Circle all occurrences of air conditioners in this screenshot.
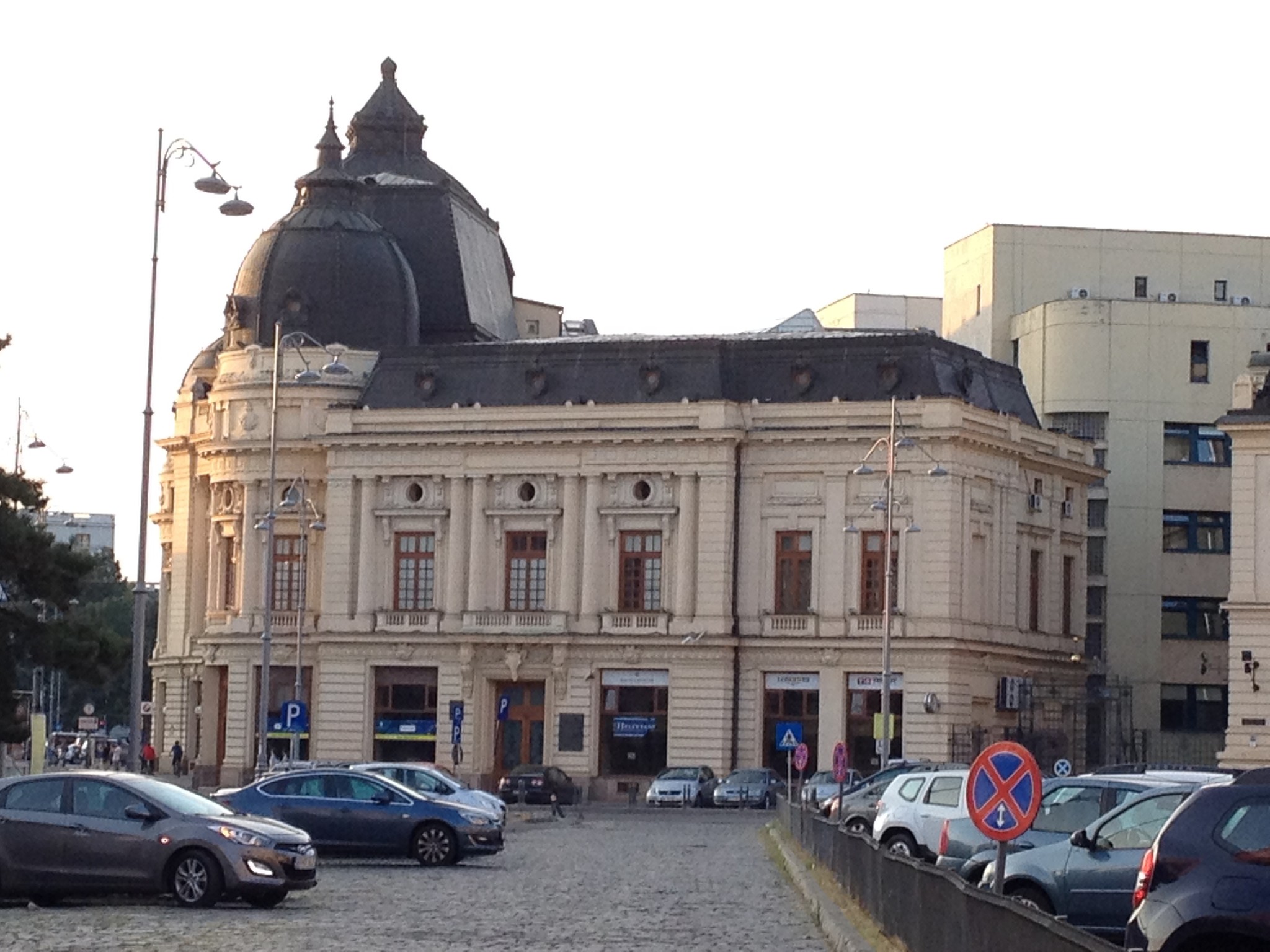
[1228,295,1253,306]
[1071,286,1090,299]
[1158,292,1178,302]
[1029,494,1043,509]
[998,675,1019,712]
[1062,501,1074,516]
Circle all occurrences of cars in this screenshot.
[871,771,971,857]
[831,779,893,837]
[984,785,1196,948]
[210,768,506,869]
[352,763,508,825]
[646,765,719,808]
[816,760,971,819]
[936,773,1162,887]
[713,768,787,809]
[801,769,865,804]
[0,770,318,909]
[496,762,580,805]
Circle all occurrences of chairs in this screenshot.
[76,790,122,817]
[318,778,354,800]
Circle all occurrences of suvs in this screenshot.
[1125,767,1270,952]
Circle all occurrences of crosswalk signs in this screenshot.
[776,722,802,750]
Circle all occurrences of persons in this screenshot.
[45,737,89,766]
[112,741,122,770]
[168,740,184,774]
[102,742,111,769]
[142,742,156,771]
[542,772,567,818]
[281,747,288,762]
[269,748,280,772]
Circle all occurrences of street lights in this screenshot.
[130,137,256,772]
[14,398,73,475]
[256,329,350,779]
[840,407,948,770]
[279,475,326,762]
